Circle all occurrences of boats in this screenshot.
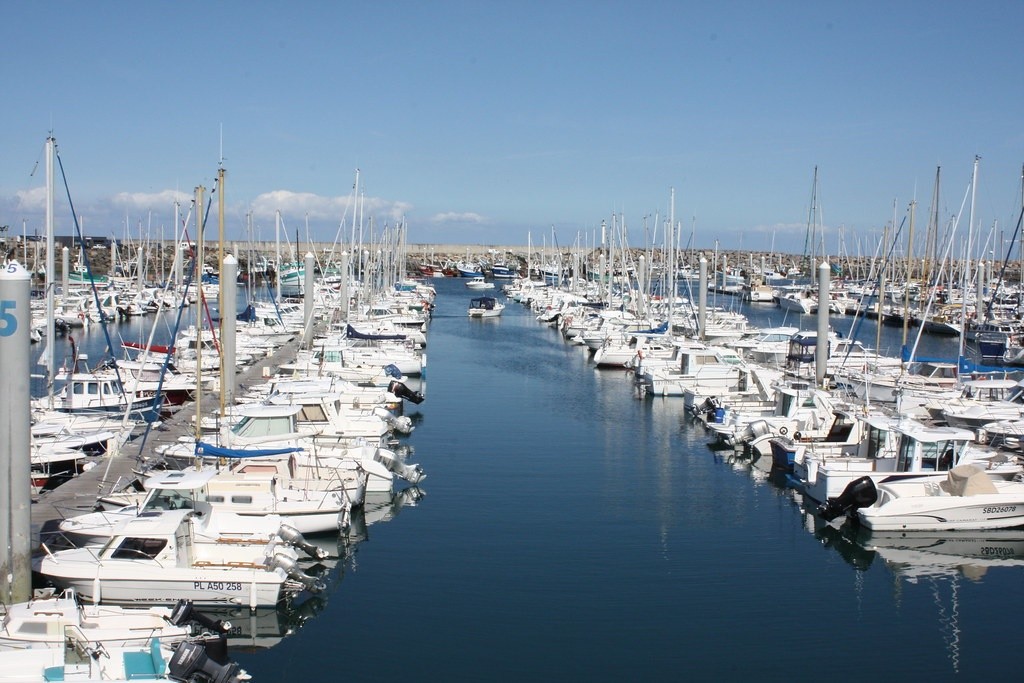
[466,276,495,289]
[0,587,252,682]
[467,296,505,320]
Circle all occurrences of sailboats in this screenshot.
[2,132,437,616]
[457,148,1024,538]
[419,246,458,278]
